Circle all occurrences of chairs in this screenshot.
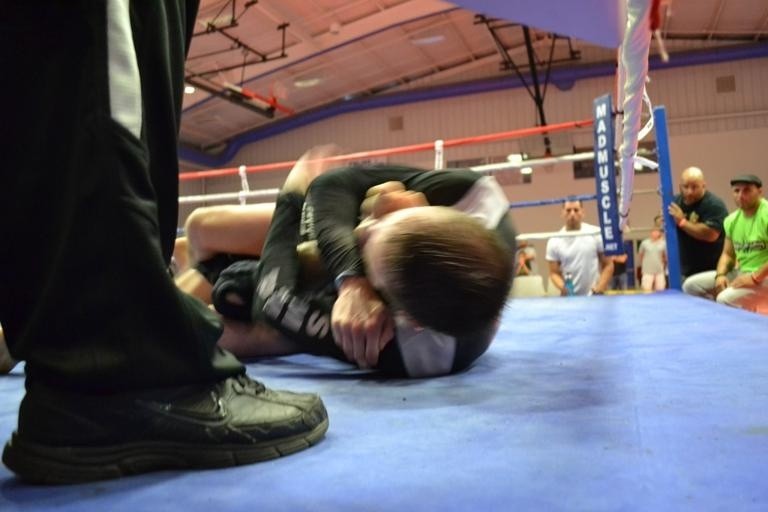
[547,274,562,295]
[509,275,544,296]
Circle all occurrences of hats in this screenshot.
[730,174,762,189]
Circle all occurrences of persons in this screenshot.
[606,249,628,290]
[680,174,768,318]
[544,194,614,296]
[514,239,538,276]
[514,251,529,276]
[638,230,667,291]
[666,166,729,297]
[0,1,329,488]
[171,143,517,381]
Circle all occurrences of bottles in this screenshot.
[565,273,573,297]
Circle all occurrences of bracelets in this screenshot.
[750,272,761,284]
[714,273,726,280]
[677,217,687,228]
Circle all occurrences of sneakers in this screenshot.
[2,367,332,486]
